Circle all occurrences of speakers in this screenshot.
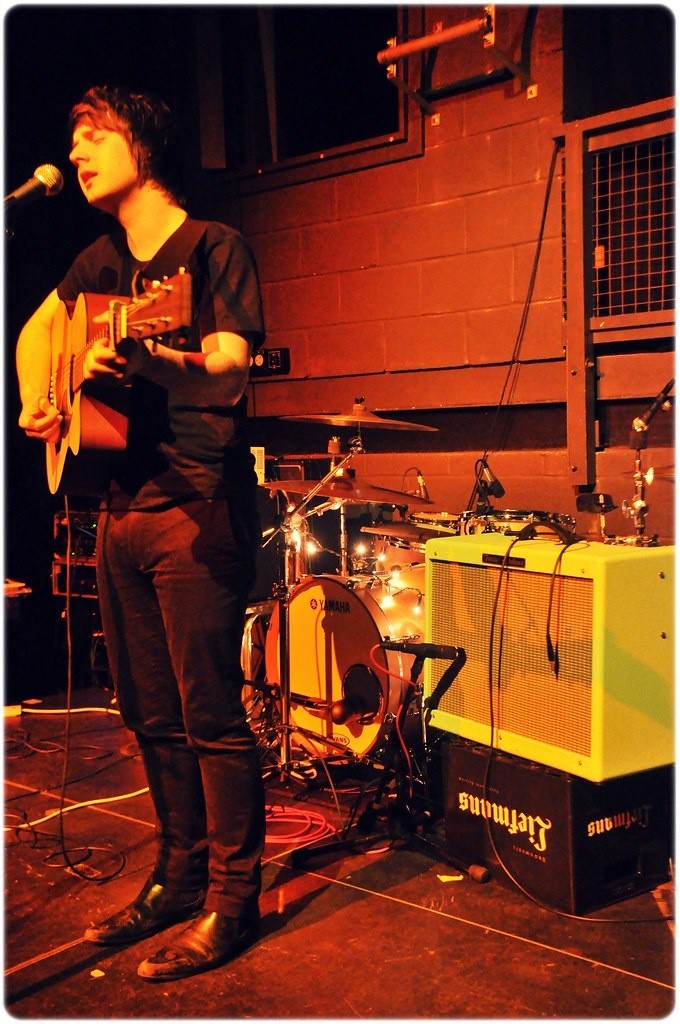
[423,532,676,783]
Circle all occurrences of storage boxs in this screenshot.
[432,732,675,917]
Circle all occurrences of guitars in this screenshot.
[43,265,196,498]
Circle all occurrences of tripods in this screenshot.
[240,419,397,791]
[290,655,489,884]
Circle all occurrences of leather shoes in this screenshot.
[85,885,207,947]
[136,910,261,981]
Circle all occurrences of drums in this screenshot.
[460,508,578,543]
[262,560,436,768]
[409,510,461,554]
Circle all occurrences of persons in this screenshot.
[13,81,268,983]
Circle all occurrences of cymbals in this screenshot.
[278,394,439,433]
[260,465,437,506]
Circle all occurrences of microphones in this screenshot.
[380,642,464,661]
[330,698,357,725]
[417,470,429,500]
[631,378,675,432]
[482,459,505,499]
[4,164,64,214]
